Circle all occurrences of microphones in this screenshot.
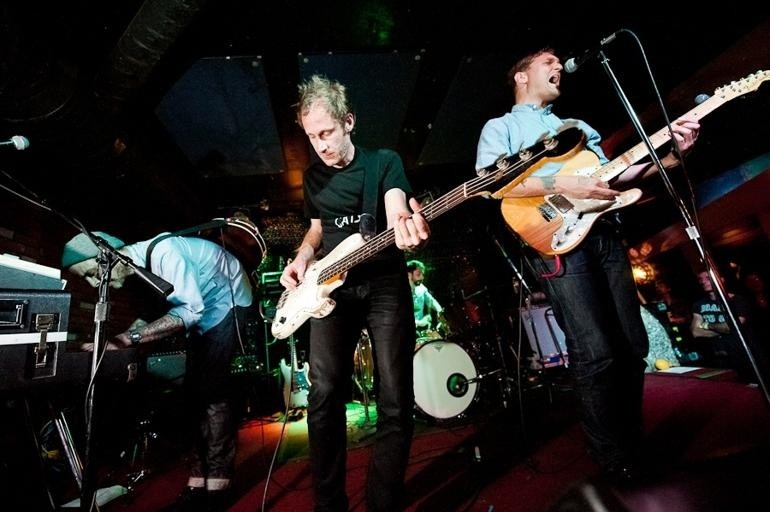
[456,375,480,391]
[564,26,622,73]
[0,132,31,154]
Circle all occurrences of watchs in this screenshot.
[128,328,143,346]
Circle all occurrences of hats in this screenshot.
[61,230,126,268]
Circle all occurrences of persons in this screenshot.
[655,278,697,351]
[279,71,438,511]
[404,258,443,336]
[684,259,757,383]
[473,45,704,500]
[60,230,257,512]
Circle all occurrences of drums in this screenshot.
[443,300,487,337]
[351,329,377,394]
[412,335,484,427]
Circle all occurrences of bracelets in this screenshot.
[669,143,693,161]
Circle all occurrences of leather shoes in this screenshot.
[174,474,239,512]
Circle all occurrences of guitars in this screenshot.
[269,124,588,341]
[501,69,770,256]
[279,334,313,410]
[191,216,267,289]
